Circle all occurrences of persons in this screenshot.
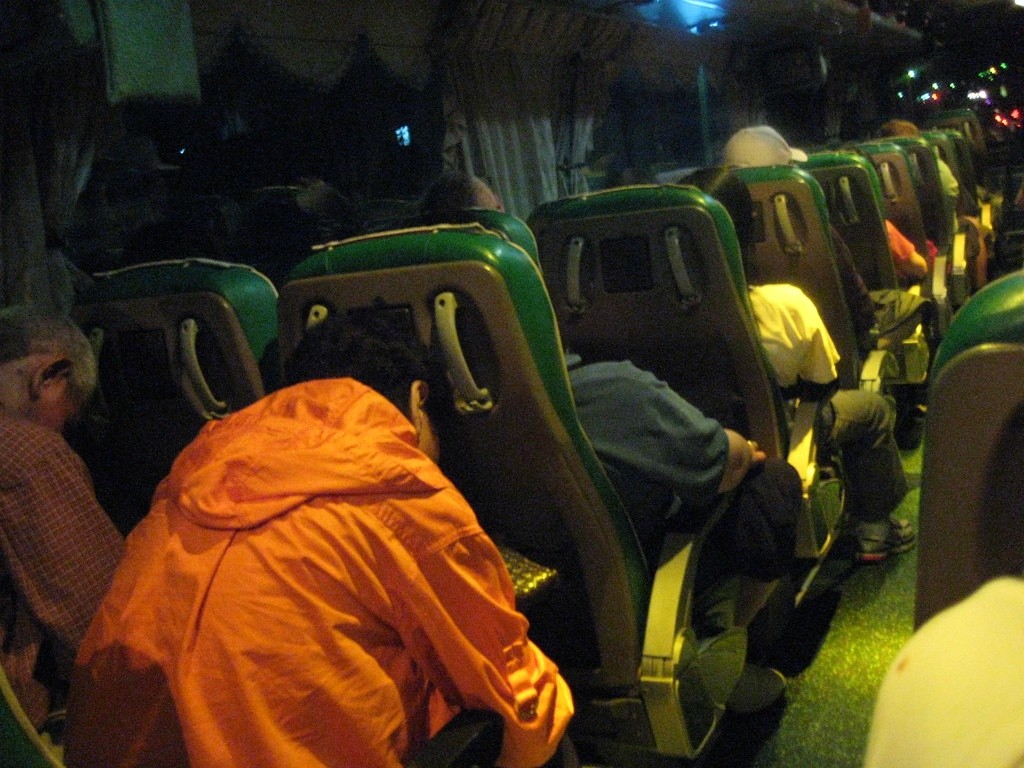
[0,297,130,744]
[62,306,580,768]
[862,397,1024,766]
[260,118,1023,715]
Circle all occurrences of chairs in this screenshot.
[0,106,1024,768]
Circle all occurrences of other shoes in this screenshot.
[851,516,917,561]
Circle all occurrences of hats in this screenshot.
[722,124,811,168]
[872,115,917,140]
